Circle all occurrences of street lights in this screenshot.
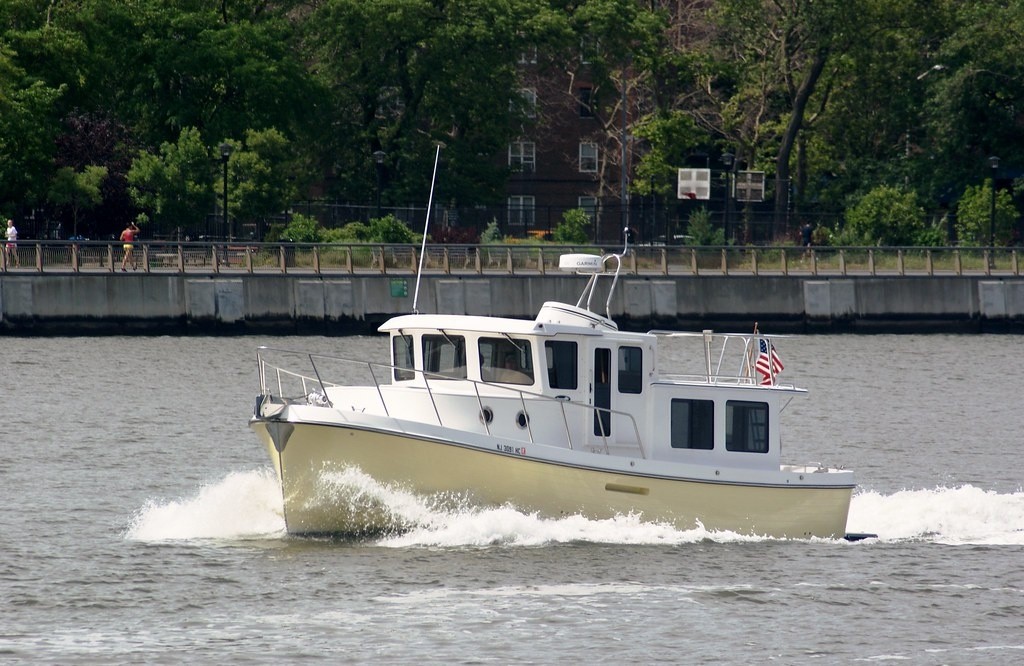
[219,142,234,241]
[988,156,1002,267]
[371,148,387,209]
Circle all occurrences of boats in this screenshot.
[250,250,858,541]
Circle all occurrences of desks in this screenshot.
[155,254,177,267]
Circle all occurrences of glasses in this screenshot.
[505,358,517,364]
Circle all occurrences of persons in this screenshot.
[480,350,530,385]
[120,221,140,272]
[799,220,819,264]
[4,220,20,269]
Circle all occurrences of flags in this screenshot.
[753,335,784,385]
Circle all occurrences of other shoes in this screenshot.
[133,262,137,270]
[120,268,127,272]
[15,264,20,268]
[6,264,10,268]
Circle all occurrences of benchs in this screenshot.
[174,251,207,266]
[218,247,259,264]
